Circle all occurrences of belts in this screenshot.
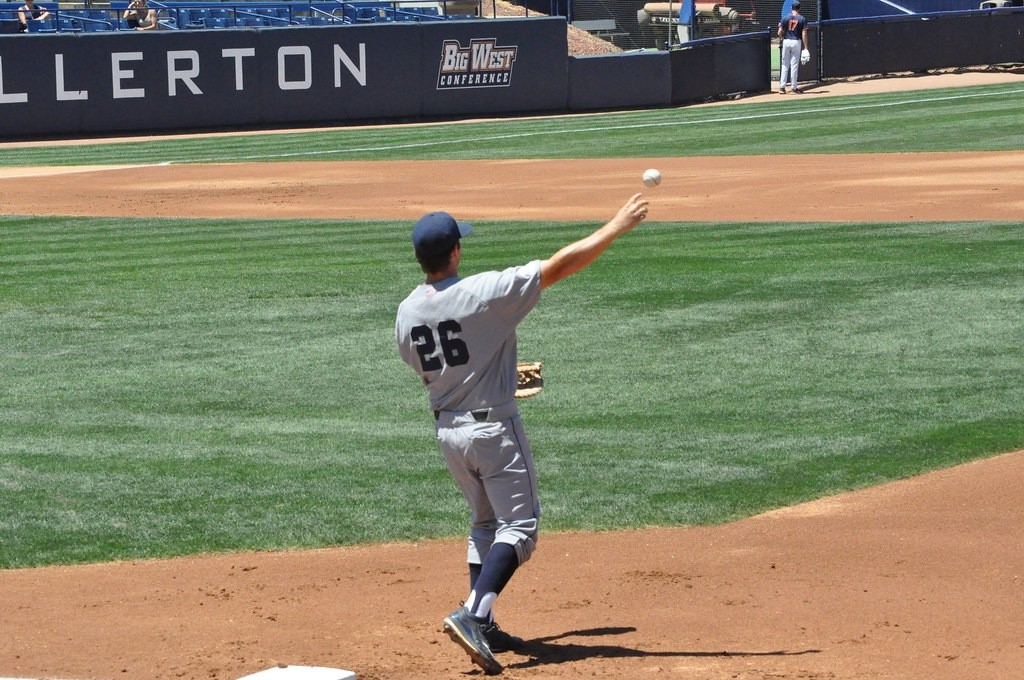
[434,411,488,421]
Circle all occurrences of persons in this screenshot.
[778,1,810,95]
[18,0,49,34]
[395,192,649,675]
[123,0,158,31]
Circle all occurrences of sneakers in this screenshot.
[480,620,524,652]
[779,88,785,94]
[443,600,502,674]
[791,88,803,94]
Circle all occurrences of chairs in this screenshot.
[0,0,483,34]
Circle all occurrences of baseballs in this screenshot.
[642,168,662,187]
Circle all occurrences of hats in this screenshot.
[792,1,800,9]
[412,211,473,257]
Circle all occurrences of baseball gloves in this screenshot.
[514,361,544,399]
[801,49,810,65]
